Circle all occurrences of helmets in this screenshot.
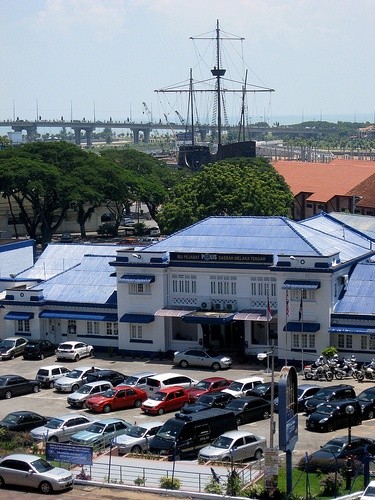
[319,356,324,359]
[334,354,338,357]
[352,355,355,357]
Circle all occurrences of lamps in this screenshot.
[289,255,296,261]
[132,253,141,259]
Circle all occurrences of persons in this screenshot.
[312,356,323,368]
[199,338,202,345]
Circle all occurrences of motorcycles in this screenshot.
[303,358,375,382]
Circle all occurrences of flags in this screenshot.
[299,294,302,321]
[266,294,272,322]
[286,290,290,316]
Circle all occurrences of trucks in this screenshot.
[149,408,238,460]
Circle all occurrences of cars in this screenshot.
[111,421,165,455]
[299,435,375,474]
[34,364,236,417]
[68,418,133,452]
[0,336,28,361]
[0,410,55,431]
[0,374,42,400]
[224,396,271,426]
[273,384,325,412]
[246,382,279,401]
[55,341,96,363]
[119,217,136,227]
[29,413,102,443]
[0,454,76,494]
[197,431,267,463]
[149,227,159,237]
[173,348,233,371]
[23,338,59,360]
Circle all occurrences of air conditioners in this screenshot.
[214,303,224,310]
[225,303,235,311]
[200,301,212,310]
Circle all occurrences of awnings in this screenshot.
[120,314,155,324]
[283,322,320,332]
[233,312,272,321]
[280,280,321,289]
[182,310,235,323]
[154,307,196,318]
[37,309,118,322]
[327,326,375,334]
[117,275,155,284]
[4,311,34,320]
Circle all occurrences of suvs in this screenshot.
[220,376,265,399]
[304,384,357,415]
[305,398,363,434]
[355,386,375,420]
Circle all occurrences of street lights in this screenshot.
[257,339,276,448]
